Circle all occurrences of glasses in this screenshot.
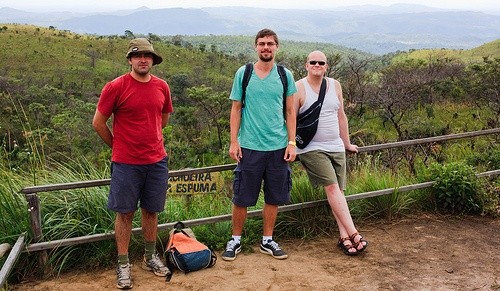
[256,43,276,47]
[308,61,326,66]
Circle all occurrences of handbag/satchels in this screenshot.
[296,99,322,149]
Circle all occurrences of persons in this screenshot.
[222,29,299,260]
[93,37,173,289]
[296,50,368,257]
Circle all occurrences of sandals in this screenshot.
[337,237,358,255]
[351,233,368,252]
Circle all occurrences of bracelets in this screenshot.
[289,141,296,146]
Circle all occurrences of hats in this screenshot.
[125,38,162,65]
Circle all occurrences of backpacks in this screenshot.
[164,222,217,273]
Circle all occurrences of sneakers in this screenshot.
[260,239,288,259]
[142,254,170,276]
[221,238,242,260]
[116,263,133,288]
